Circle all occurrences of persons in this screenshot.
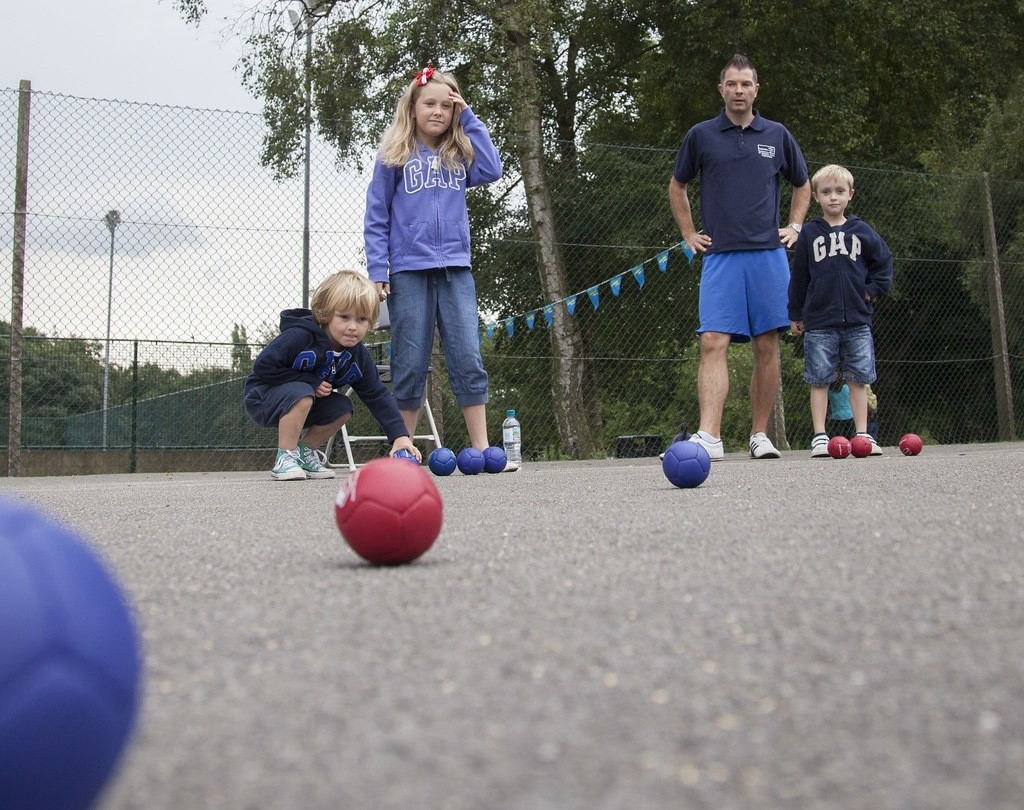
[659,54,811,460]
[787,164,894,457]
[827,361,879,442]
[365,69,519,472]
[243,270,422,479]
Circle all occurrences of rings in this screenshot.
[787,236,790,239]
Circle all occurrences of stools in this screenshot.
[323,365,442,472]
[615,435,665,458]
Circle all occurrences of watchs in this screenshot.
[789,223,801,234]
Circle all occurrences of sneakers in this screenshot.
[658,433,725,462]
[811,434,833,458]
[500,458,519,472]
[855,433,884,456]
[300,446,338,479]
[748,435,782,460]
[270,446,307,481]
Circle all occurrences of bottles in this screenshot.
[501,410,522,468]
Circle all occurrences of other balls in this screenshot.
[662,440,711,489]
[428,447,457,476]
[482,446,507,473]
[457,447,486,475]
[898,433,923,456]
[334,455,443,566]
[392,449,421,467]
[1,489,146,810]
[827,435,852,459]
[849,435,873,458]
